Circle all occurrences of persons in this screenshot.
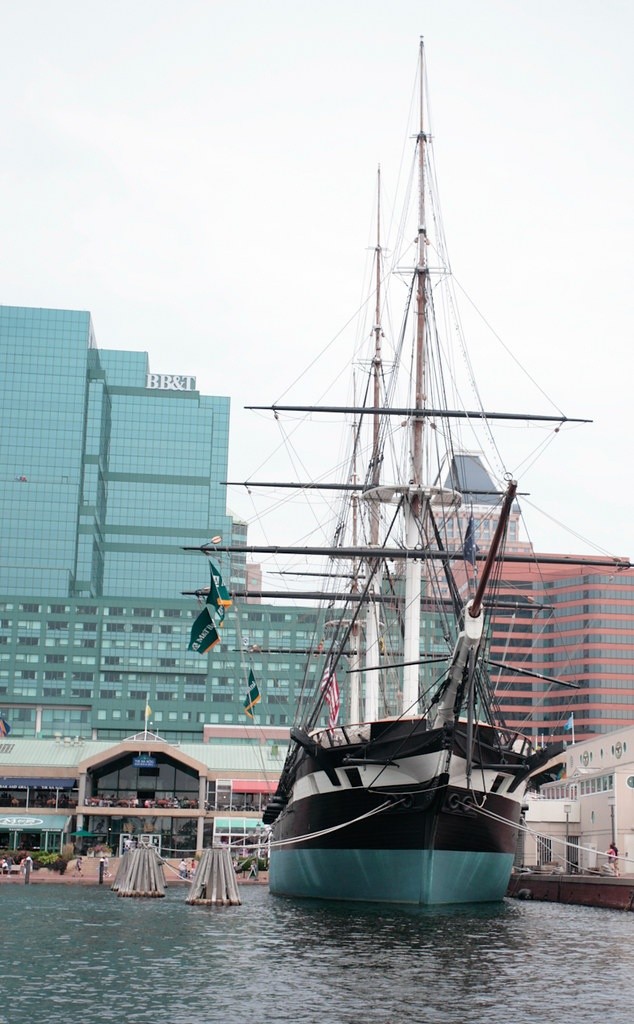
[0,789,269,881]
[605,842,621,877]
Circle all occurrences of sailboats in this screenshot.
[177,35,633,907]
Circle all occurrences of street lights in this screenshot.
[562,803,574,875]
[607,794,617,845]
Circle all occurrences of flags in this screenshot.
[187,607,220,655]
[564,716,572,730]
[243,667,260,719]
[320,654,340,727]
[205,560,232,626]
